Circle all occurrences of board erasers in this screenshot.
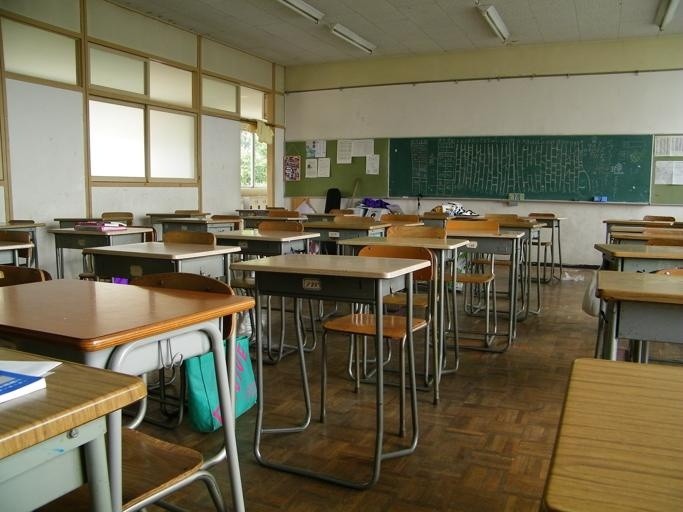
[508,193,524,201]
[593,195,607,202]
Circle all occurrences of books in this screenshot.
[74,226,128,233]
[351,201,390,221]
[76,222,120,227]
[0,369,48,404]
[88,219,127,226]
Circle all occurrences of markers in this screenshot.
[403,196,408,198]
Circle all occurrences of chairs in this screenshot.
[643,215,675,222]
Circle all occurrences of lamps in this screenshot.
[276,0,325,25]
[476,4,511,43]
[652,0,680,32]
[329,22,376,57]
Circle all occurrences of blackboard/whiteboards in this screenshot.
[387,134,654,206]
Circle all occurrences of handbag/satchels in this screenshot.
[582,270,607,317]
[184,335,258,433]
